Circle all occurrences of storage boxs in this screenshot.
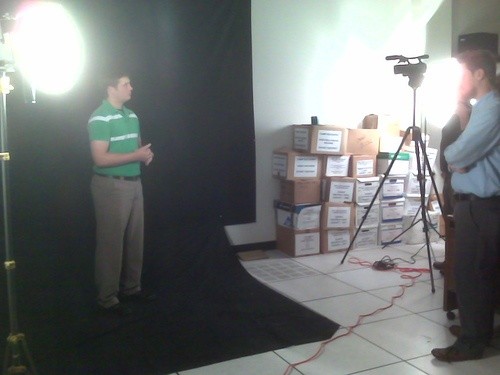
[273,123,448,256]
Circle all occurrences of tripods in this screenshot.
[341,81,444,292]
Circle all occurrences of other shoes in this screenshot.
[93,301,133,316]
[432,346,484,361]
[119,290,156,303]
[449,324,492,345]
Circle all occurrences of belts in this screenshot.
[94,172,141,182]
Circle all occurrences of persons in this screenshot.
[430,46,500,367]
[85,67,156,315]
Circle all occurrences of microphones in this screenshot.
[386,56,403,60]
